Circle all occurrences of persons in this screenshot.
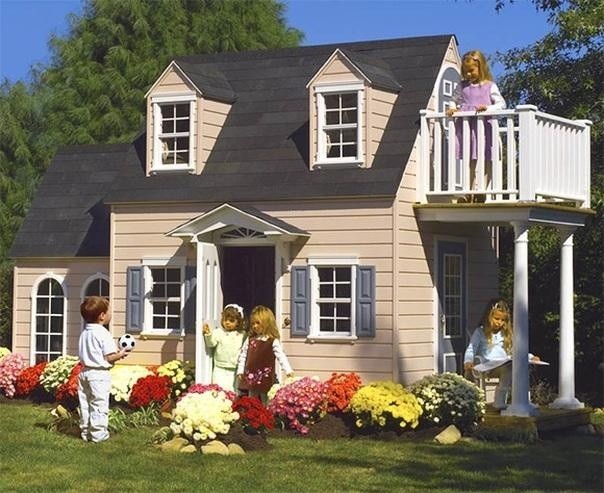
[77,295,129,444]
[448,50,507,202]
[236,305,294,406]
[202,304,246,396]
[464,297,540,411]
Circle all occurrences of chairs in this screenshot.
[467,327,511,405]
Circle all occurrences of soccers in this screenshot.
[117,334,135,351]
